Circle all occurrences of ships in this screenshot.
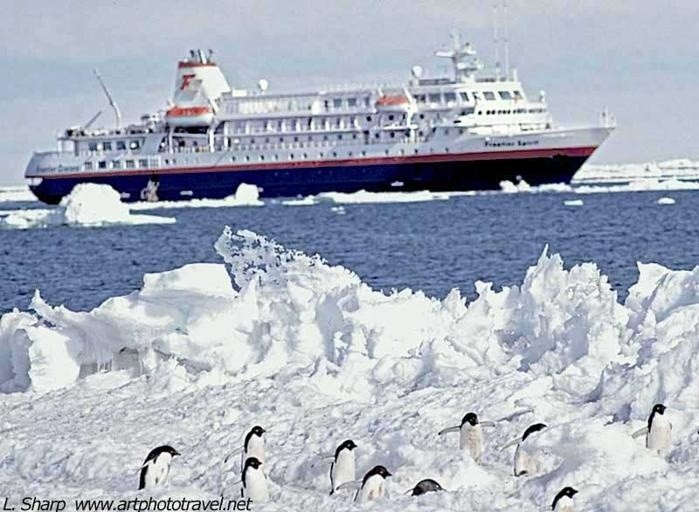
[21,33,617,203]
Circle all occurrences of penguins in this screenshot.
[134,445,181,493]
[240,457,268,503]
[224,426,266,473]
[324,439,358,496]
[551,486,578,512]
[402,478,445,497]
[499,423,547,477]
[438,412,496,465]
[354,465,392,506]
[631,403,672,455]
